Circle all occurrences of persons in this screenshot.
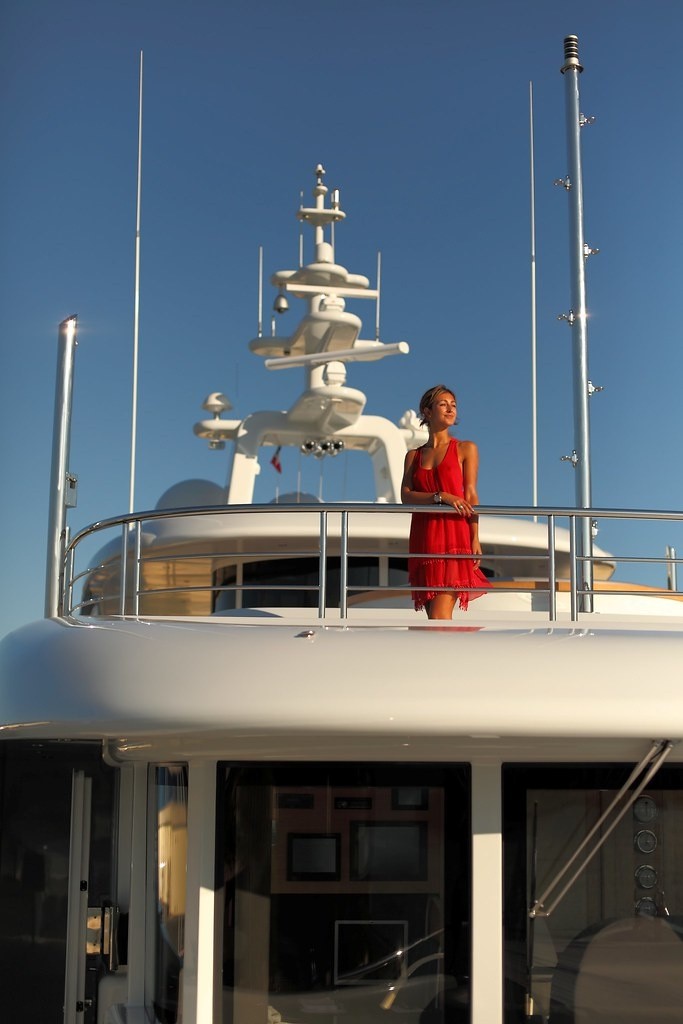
[400,384,494,621]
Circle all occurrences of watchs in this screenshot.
[433,490,443,504]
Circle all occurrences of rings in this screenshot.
[458,505,463,509]
